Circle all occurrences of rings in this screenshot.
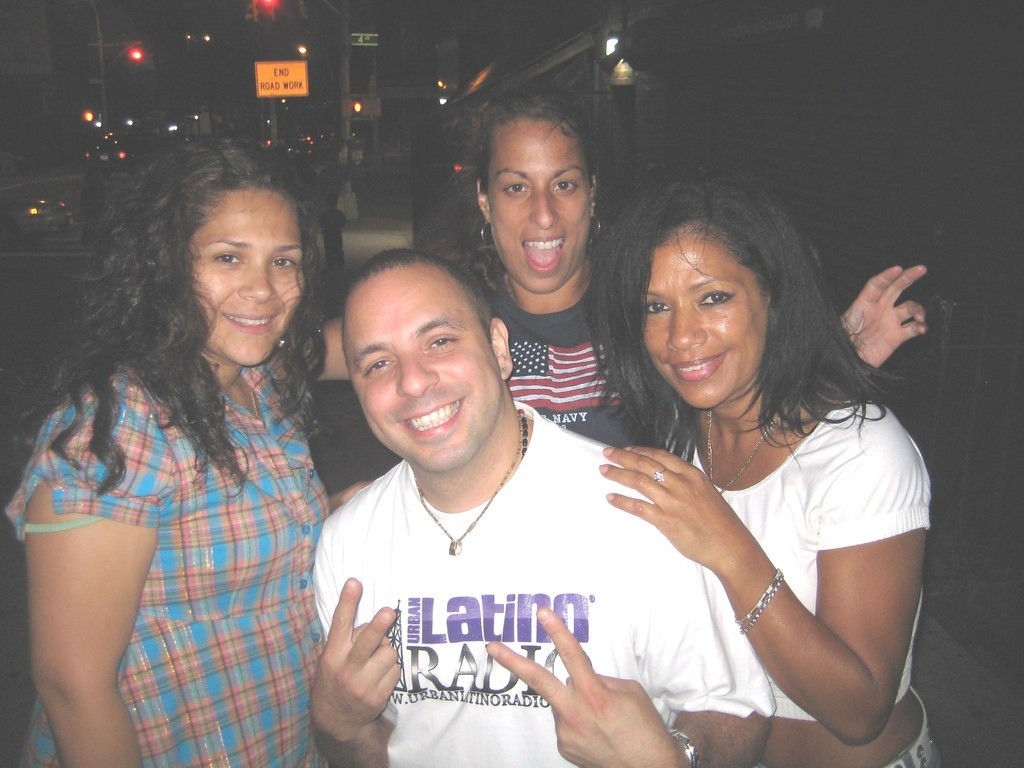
[652,469,667,483]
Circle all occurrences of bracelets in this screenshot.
[735,568,784,635]
[668,728,700,768]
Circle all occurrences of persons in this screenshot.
[4,136,379,768]
[303,87,928,448]
[600,175,942,768]
[309,250,774,768]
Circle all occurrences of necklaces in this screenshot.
[706,410,779,495]
[415,410,528,558]
[240,376,259,418]
